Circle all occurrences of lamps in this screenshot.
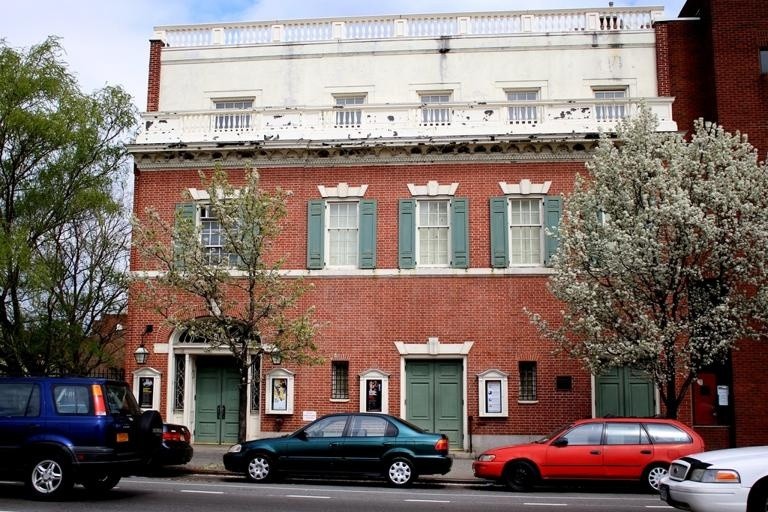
[133,322,154,367]
[271,328,285,368]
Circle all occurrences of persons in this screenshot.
[369,381,378,396]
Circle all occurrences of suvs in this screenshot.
[0,376,166,498]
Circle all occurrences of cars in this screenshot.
[656,443,768,512]
[55,394,193,475]
[222,412,452,489]
[466,416,703,495]
